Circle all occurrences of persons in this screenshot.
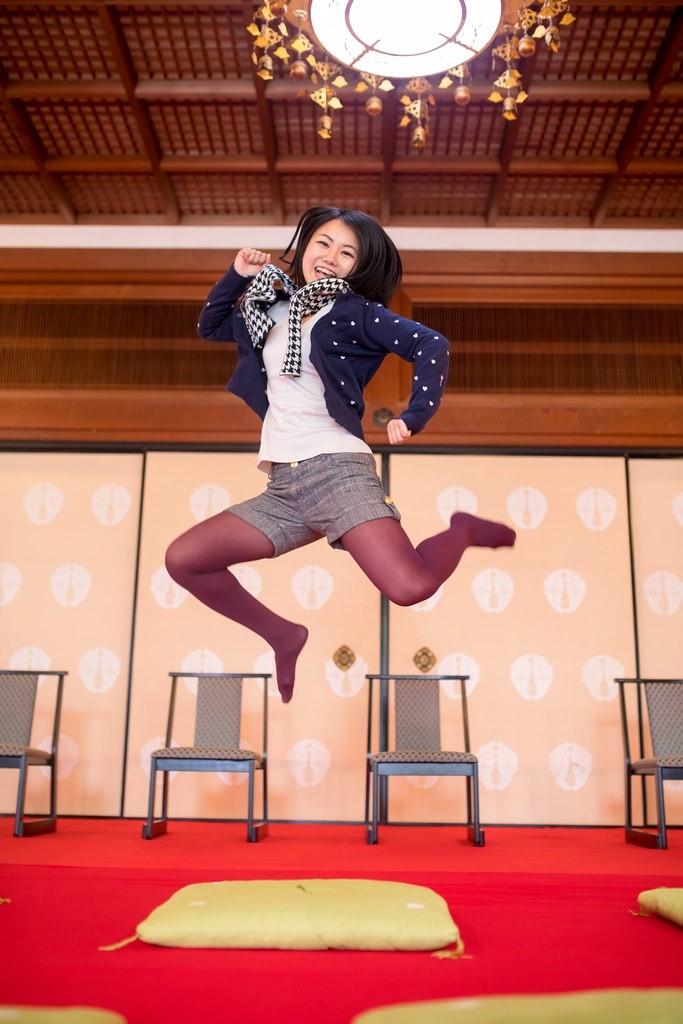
[164,207,515,703]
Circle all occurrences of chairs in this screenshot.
[141,671,274,843]
[0,670,69,838]
[611,678,683,850]
[362,674,486,849]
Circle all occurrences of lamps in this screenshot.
[248,0,576,153]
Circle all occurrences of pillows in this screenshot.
[135,878,465,960]
